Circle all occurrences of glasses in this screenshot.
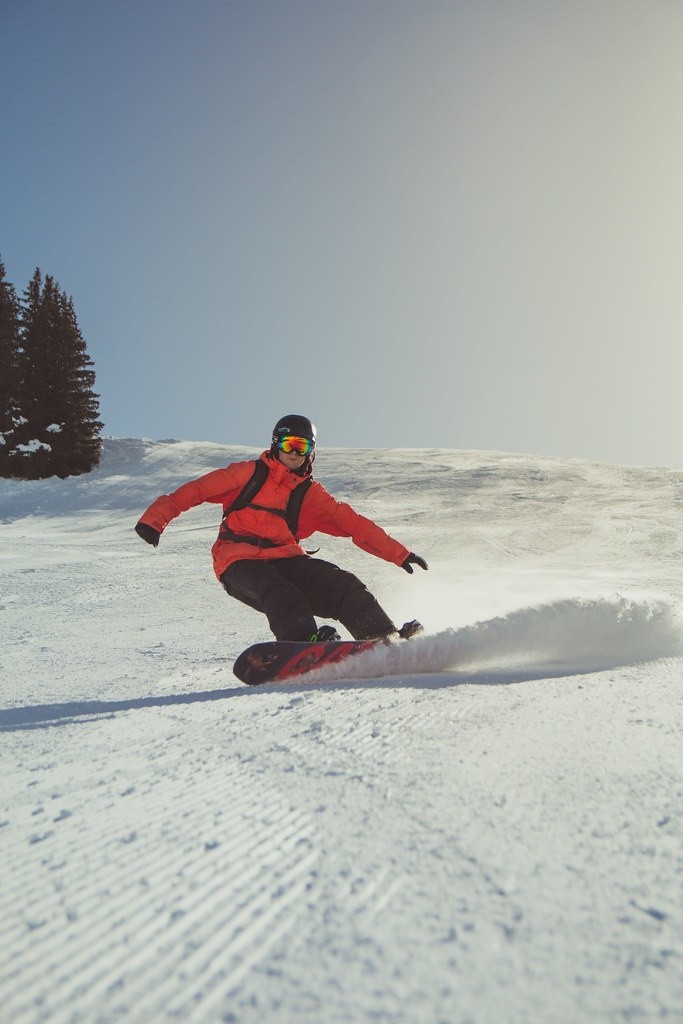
[274,436,315,455]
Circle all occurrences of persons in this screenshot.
[134,415,428,641]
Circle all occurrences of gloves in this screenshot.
[401,552,428,574]
[135,522,161,547]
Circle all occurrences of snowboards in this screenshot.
[231,638,464,685]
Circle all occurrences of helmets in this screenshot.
[272,414,316,476]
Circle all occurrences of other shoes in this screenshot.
[311,626,341,641]
[397,620,423,640]
